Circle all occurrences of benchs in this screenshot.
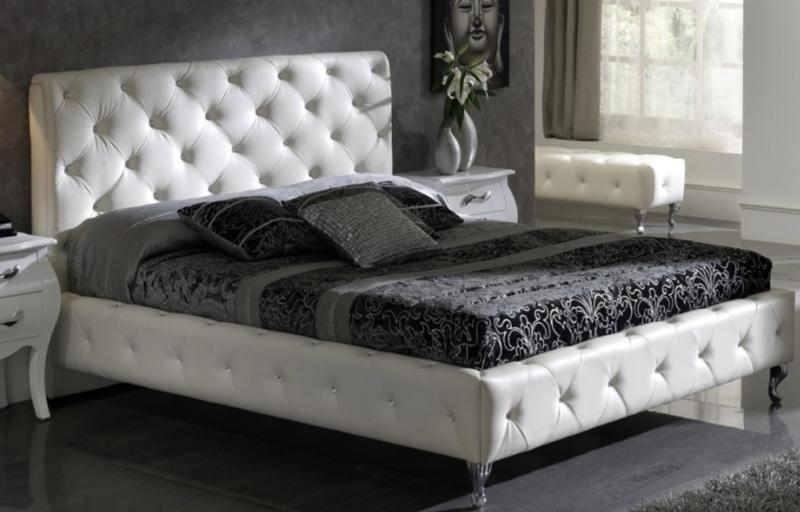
[532,145,687,235]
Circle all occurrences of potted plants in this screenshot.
[431,44,499,176]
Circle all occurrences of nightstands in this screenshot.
[393,165,520,229]
[0,231,66,420]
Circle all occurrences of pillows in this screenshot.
[175,180,464,271]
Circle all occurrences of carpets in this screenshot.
[0,381,800,512]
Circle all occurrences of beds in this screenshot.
[24,48,796,512]
